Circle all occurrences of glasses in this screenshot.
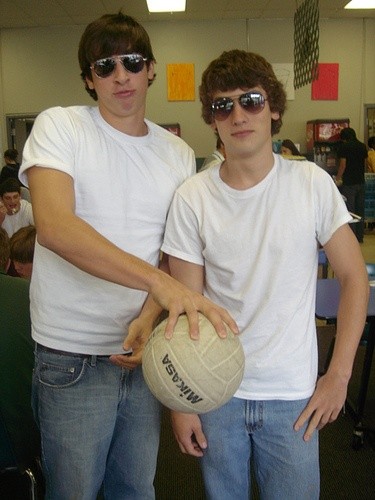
[211,92,268,121]
[89,54,148,79]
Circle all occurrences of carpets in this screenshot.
[151,323,374,500]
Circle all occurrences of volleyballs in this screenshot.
[140,312,246,415]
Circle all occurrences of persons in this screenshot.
[367,136,375,173]
[0,149,25,187]
[335,127,368,243]
[199,136,226,173]
[281,139,301,155]
[9,225,37,279]
[0,227,40,466]
[17,12,241,500]
[159,48,369,500]
[0,179,35,238]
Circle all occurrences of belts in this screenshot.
[36,343,132,359]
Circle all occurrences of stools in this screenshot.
[0,462,43,500]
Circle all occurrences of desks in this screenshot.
[316,277,375,449]
